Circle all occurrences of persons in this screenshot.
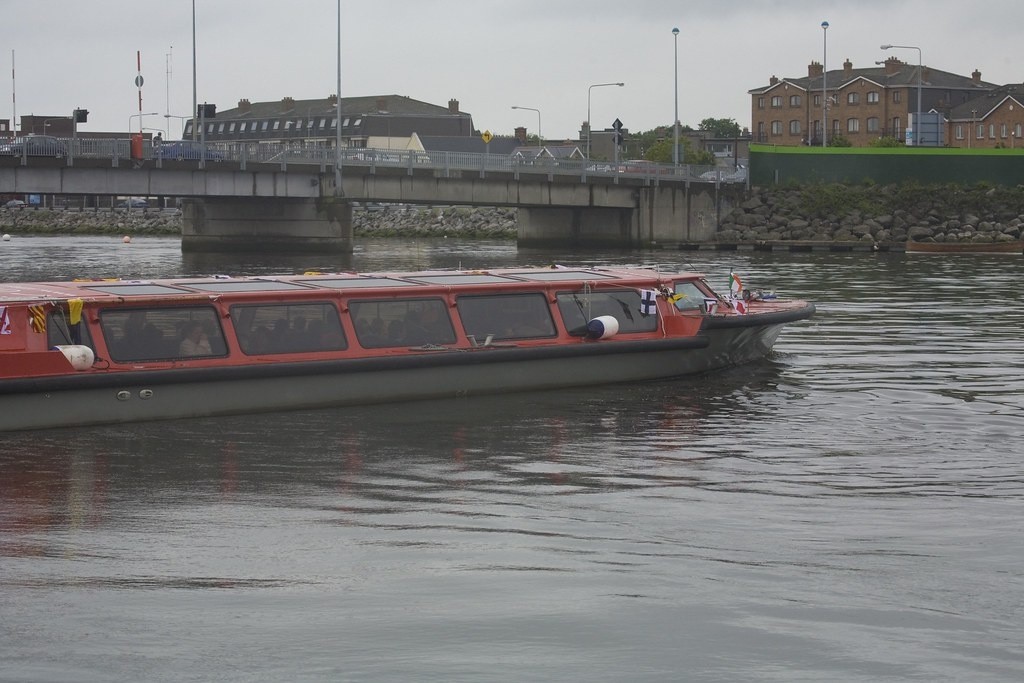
[153,132,162,155]
[105,307,435,362]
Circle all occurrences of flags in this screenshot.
[0,306,12,335]
[68,300,83,326]
[666,288,746,315]
[27,304,46,333]
[641,290,657,314]
[728,272,742,292]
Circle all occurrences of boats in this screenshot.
[0,263,819,428]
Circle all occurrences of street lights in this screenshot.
[821,21,829,147]
[880,45,922,148]
[511,105,542,154]
[163,114,194,142]
[128,112,159,139]
[43,115,72,136]
[672,27,679,172]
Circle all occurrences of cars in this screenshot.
[587,82,625,167]
[0,134,68,156]
[619,160,668,174]
[153,141,223,162]
